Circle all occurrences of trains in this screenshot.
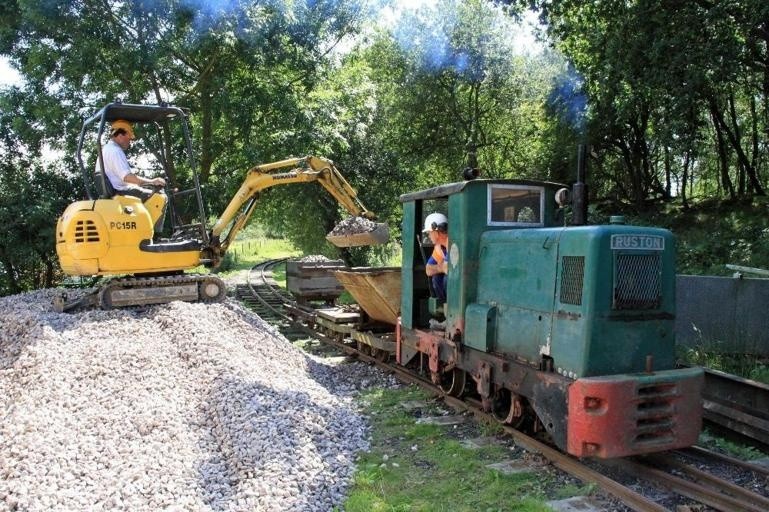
[286,143,706,459]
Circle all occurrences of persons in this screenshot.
[95,119,168,243]
[423,210,448,300]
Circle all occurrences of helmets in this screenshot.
[111,119,134,139]
[422,211,448,233]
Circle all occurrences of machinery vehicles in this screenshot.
[52,97,390,313]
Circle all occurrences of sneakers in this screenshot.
[153,235,168,244]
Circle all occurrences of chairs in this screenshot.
[94,173,148,204]
